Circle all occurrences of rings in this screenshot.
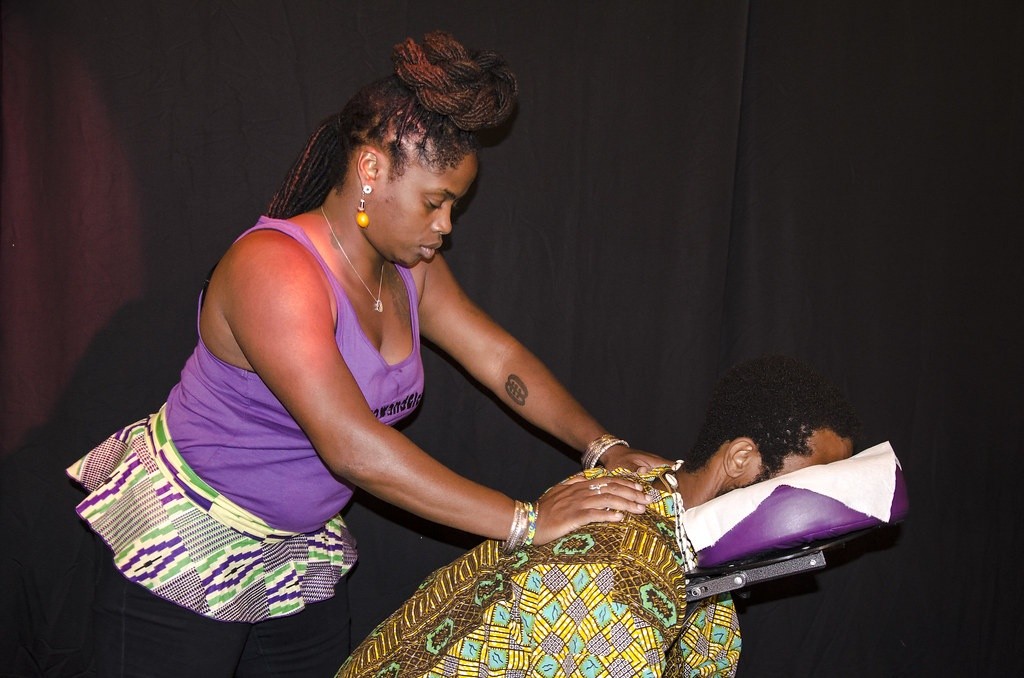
[589,483,608,493]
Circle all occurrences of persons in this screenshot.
[334,356,860,678]
[66,31,680,678]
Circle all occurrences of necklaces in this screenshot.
[660,457,696,577]
[319,204,383,313]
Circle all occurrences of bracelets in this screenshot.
[503,498,540,556]
[581,434,629,469]
